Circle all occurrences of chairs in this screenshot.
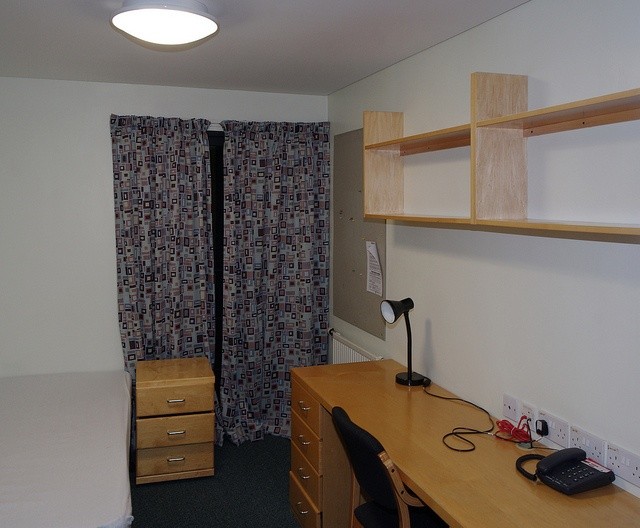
[330,406,448,528]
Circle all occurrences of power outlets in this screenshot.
[518,399,535,430]
[606,441,640,488]
[570,423,606,467]
[501,393,518,422]
[537,409,569,448]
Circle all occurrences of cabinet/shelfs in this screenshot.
[362,70,640,241]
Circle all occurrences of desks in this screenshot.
[288,358,640,528]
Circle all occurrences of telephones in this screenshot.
[516,448,616,495]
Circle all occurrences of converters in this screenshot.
[535,419,548,436]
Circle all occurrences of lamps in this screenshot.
[379,297,424,386]
[108,0,220,55]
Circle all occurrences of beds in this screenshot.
[1,370,136,528]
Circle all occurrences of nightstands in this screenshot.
[134,357,216,485]
